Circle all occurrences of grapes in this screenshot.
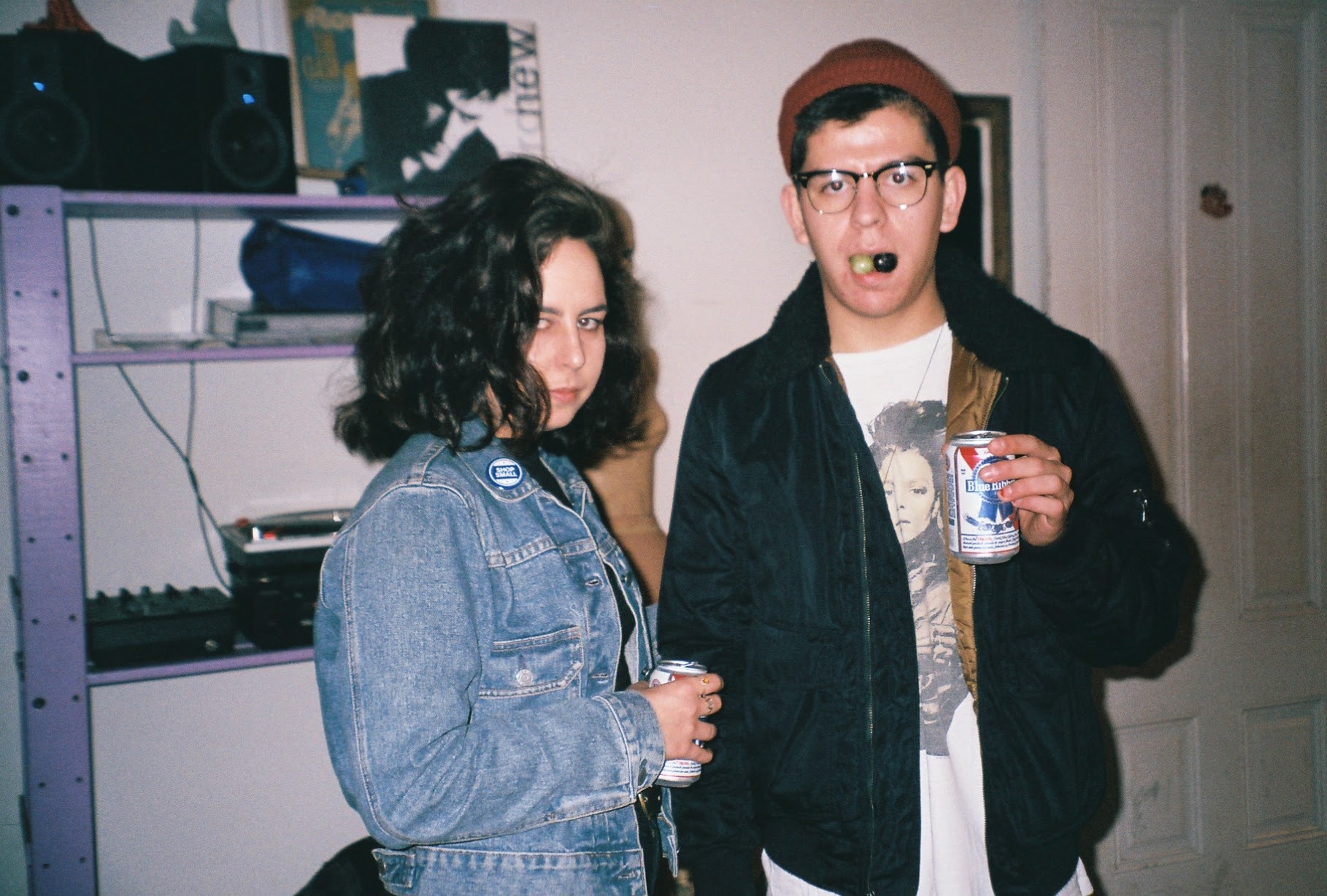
[849,251,896,274]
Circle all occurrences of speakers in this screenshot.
[0,28,300,196]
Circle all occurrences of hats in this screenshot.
[777,39,963,184]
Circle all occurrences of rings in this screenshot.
[705,696,713,713]
[700,674,709,693]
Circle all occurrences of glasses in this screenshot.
[792,160,944,215]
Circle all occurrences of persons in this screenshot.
[314,155,724,895]
[656,38,1189,896]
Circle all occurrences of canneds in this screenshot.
[944,431,1019,565]
[649,661,708,787]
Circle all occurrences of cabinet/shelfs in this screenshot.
[0,186,449,896]
